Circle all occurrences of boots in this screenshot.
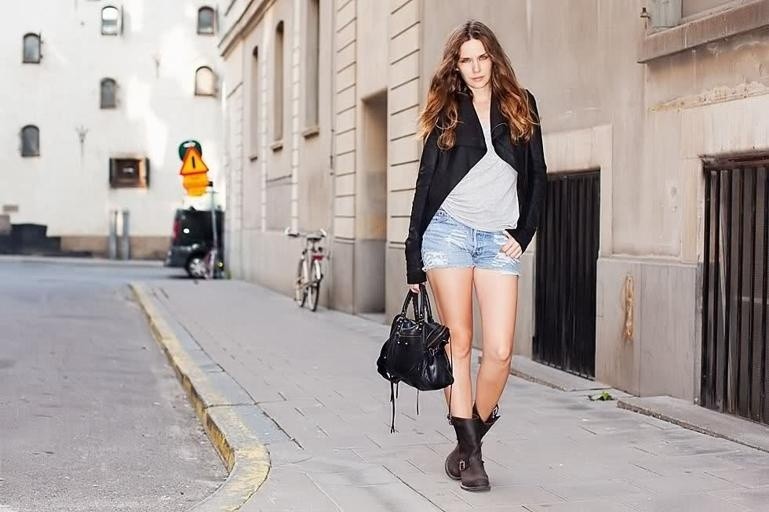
[443,404,502,492]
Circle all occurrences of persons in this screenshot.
[402,19,549,492]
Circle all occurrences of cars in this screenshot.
[163,206,224,279]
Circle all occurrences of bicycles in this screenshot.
[285,224,328,313]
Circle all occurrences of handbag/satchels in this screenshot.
[378,284,455,393]
